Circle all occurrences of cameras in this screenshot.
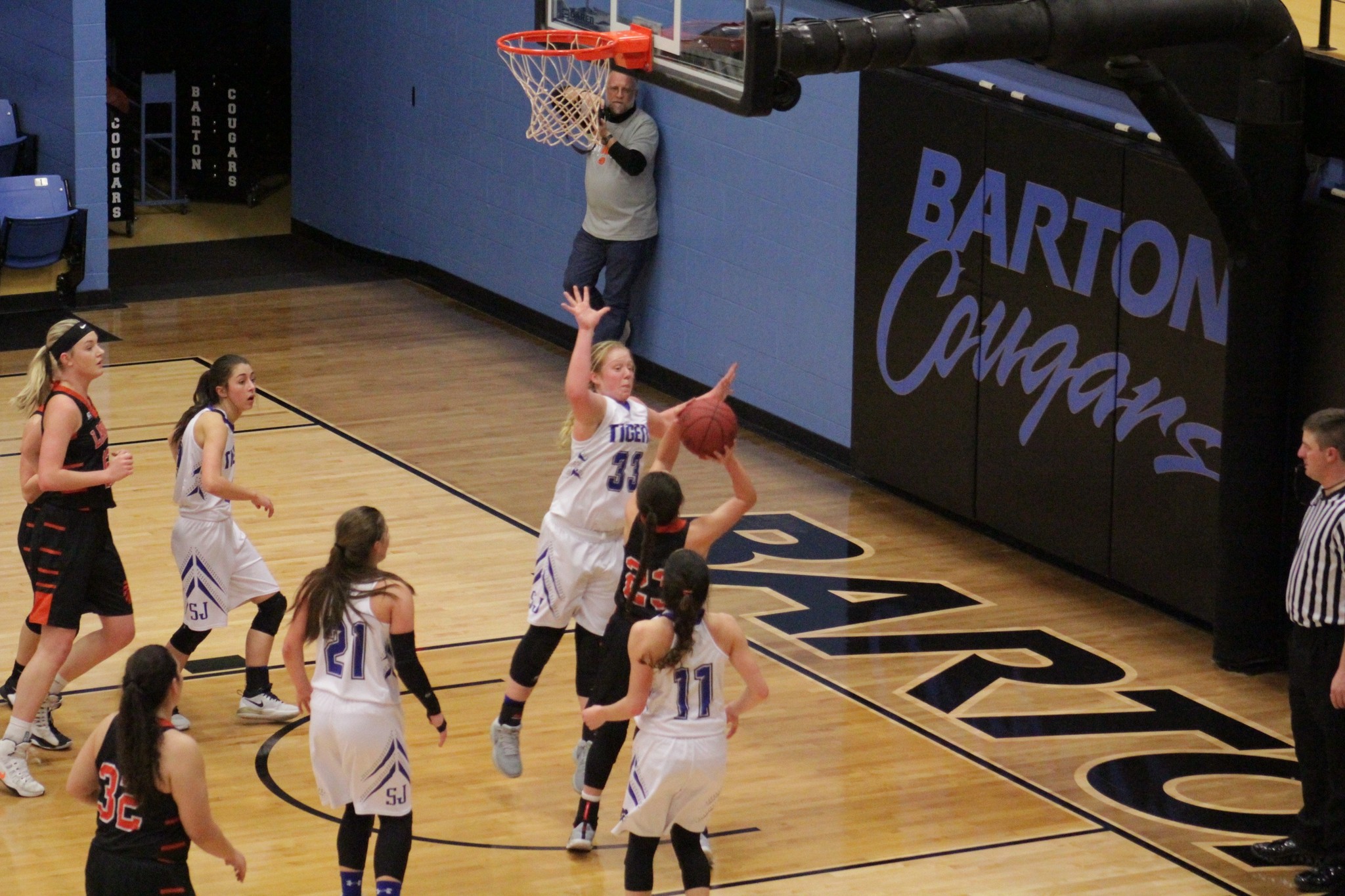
[598,107,605,121]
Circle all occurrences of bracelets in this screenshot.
[577,90,587,102]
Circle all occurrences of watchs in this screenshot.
[601,134,613,145]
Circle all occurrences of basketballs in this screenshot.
[679,398,738,460]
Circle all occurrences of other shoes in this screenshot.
[619,319,632,346]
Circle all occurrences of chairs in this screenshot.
[0,94,38,175]
[0,174,78,268]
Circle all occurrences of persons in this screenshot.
[1251,407,1345,896]
[0,319,136,797]
[165,355,300,731]
[492,285,738,779]
[66,645,246,896]
[282,507,447,896]
[580,549,769,896]
[551,70,658,348]
[580,0,593,14]
[566,409,757,853]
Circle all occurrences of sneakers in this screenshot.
[236,683,300,722]
[0,685,16,710]
[491,716,522,778]
[566,821,598,853]
[7,692,73,750]
[0,738,45,797]
[170,706,191,730]
[699,832,715,864]
[572,738,593,794]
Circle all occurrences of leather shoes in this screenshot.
[1250,838,1322,867]
[1294,865,1345,893]
